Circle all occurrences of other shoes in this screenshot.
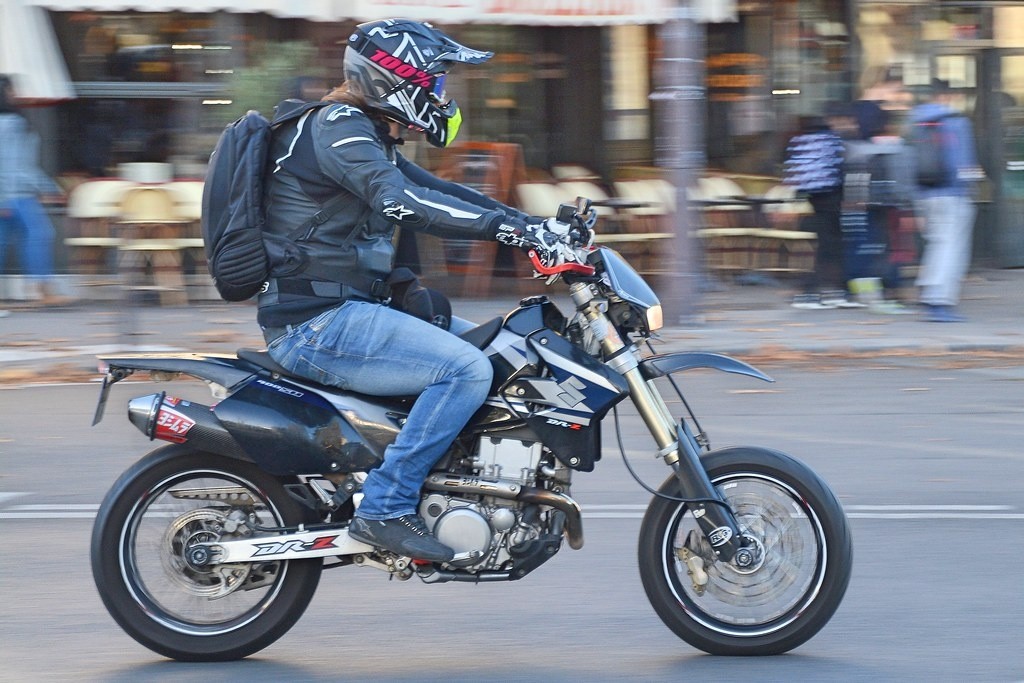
[923,301,969,322]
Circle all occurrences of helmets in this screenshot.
[343,17,496,148]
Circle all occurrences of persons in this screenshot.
[797,59,996,324]
[256,18,582,564]
[2,73,78,318]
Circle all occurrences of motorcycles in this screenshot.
[89,196,854,662]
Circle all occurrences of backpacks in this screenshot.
[203,102,360,300]
[907,112,967,191]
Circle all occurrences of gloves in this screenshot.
[494,216,576,286]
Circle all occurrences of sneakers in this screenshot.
[347,513,455,563]
